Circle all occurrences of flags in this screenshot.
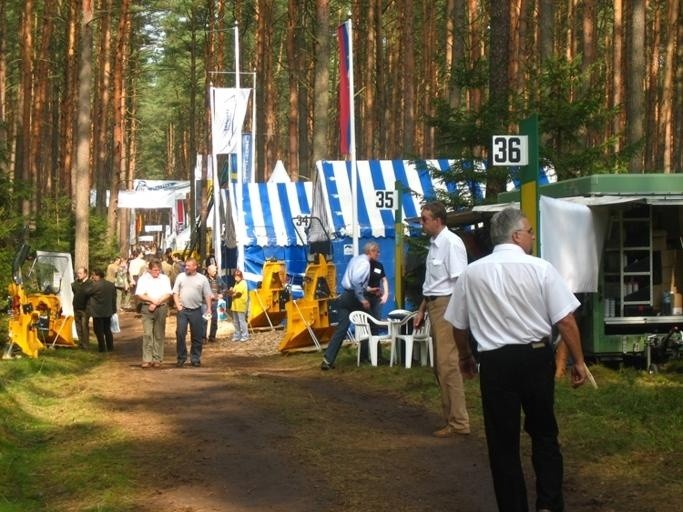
[335,21,353,157]
[174,86,256,234]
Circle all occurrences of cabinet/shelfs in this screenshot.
[602,201,654,317]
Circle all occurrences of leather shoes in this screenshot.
[432,425,470,438]
[193,362,200,367]
[141,362,151,368]
[320,365,334,370]
[176,360,184,367]
[153,363,159,368]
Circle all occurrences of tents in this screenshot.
[302,153,560,344]
[219,181,314,320]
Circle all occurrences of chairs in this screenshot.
[348,309,395,368]
[389,311,434,370]
[387,307,411,314]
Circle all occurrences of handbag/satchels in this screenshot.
[110,313,119,333]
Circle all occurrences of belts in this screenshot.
[185,306,200,312]
[143,304,164,307]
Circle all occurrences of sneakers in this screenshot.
[239,337,247,342]
[231,338,239,341]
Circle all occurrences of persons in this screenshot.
[224,270,250,342]
[103,243,222,313]
[201,261,222,344]
[439,206,587,511]
[412,200,471,438]
[133,261,173,369]
[319,238,380,371]
[368,258,390,364]
[171,257,214,369]
[82,267,117,353]
[70,267,92,348]
